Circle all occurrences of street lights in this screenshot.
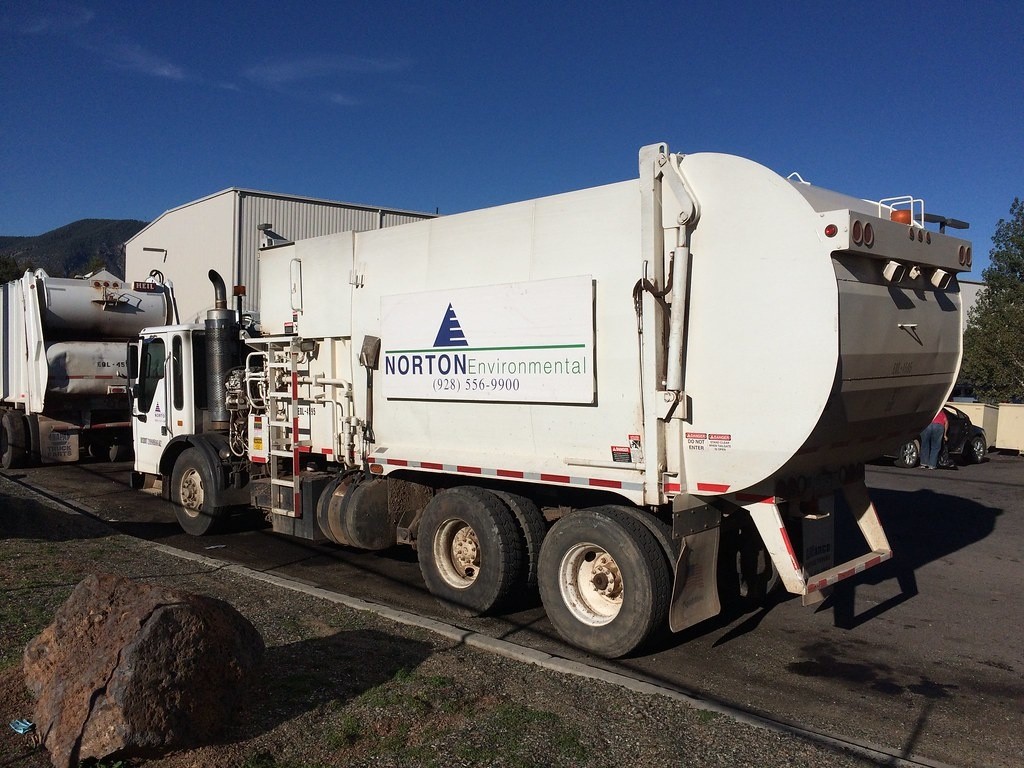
[916,213,970,235]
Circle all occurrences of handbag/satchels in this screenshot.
[936,438,958,470]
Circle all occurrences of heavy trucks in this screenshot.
[0,267,167,470]
[126,142,972,657]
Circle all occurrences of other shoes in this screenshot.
[918,463,937,471]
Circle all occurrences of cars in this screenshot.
[881,405,987,468]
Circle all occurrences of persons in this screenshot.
[920,410,948,469]
